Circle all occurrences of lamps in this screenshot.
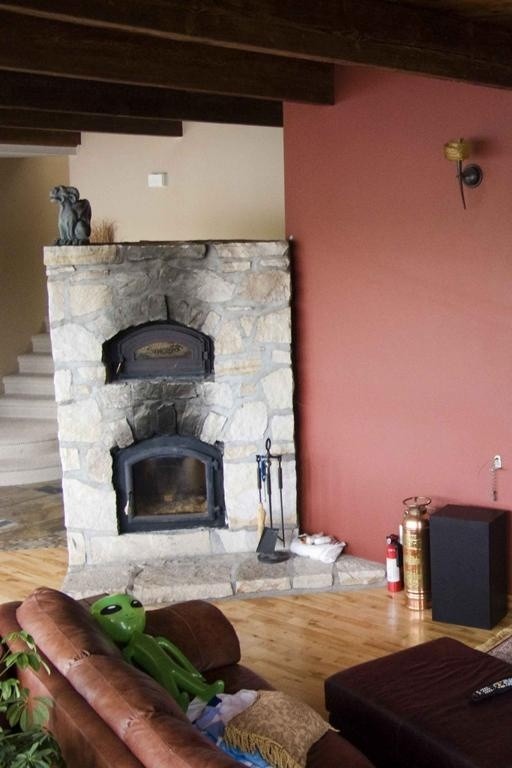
[443,137,483,209]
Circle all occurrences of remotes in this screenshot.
[470,676,511,703]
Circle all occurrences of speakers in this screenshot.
[428,503,508,630]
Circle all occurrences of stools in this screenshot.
[324,636,512,765]
[0,585,375,767]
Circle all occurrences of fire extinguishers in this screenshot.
[385,496,431,599]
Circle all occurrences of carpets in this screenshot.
[474,623,512,663]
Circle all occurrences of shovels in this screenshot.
[256,438,279,553]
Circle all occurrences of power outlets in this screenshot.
[494,455,502,471]
